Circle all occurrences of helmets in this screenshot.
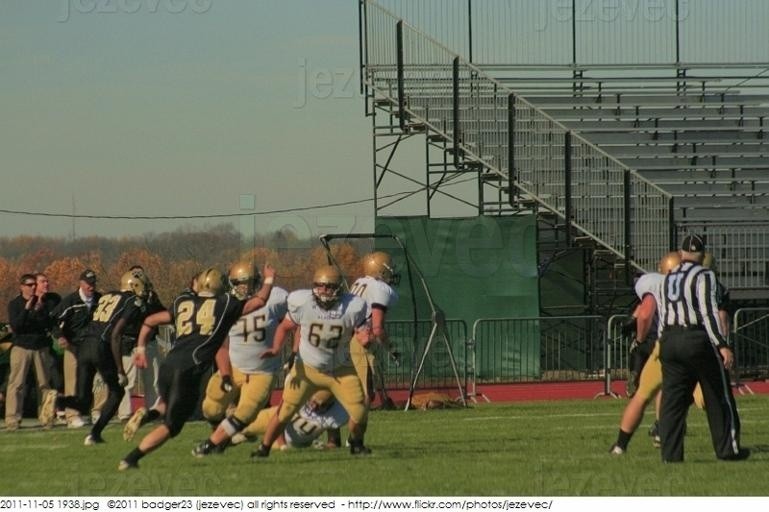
[197,261,260,297]
[656,233,715,274]
[120,270,154,299]
[311,251,396,310]
[304,390,335,417]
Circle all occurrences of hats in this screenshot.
[80,269,96,283]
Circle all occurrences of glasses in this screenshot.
[25,283,37,286]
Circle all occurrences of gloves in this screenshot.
[622,317,645,353]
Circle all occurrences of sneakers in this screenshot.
[724,448,750,460]
[6,389,108,446]
[118,406,149,471]
[192,439,270,458]
[346,433,371,455]
[608,420,684,463]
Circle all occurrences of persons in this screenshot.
[610,234,751,464]
[6,252,399,472]
